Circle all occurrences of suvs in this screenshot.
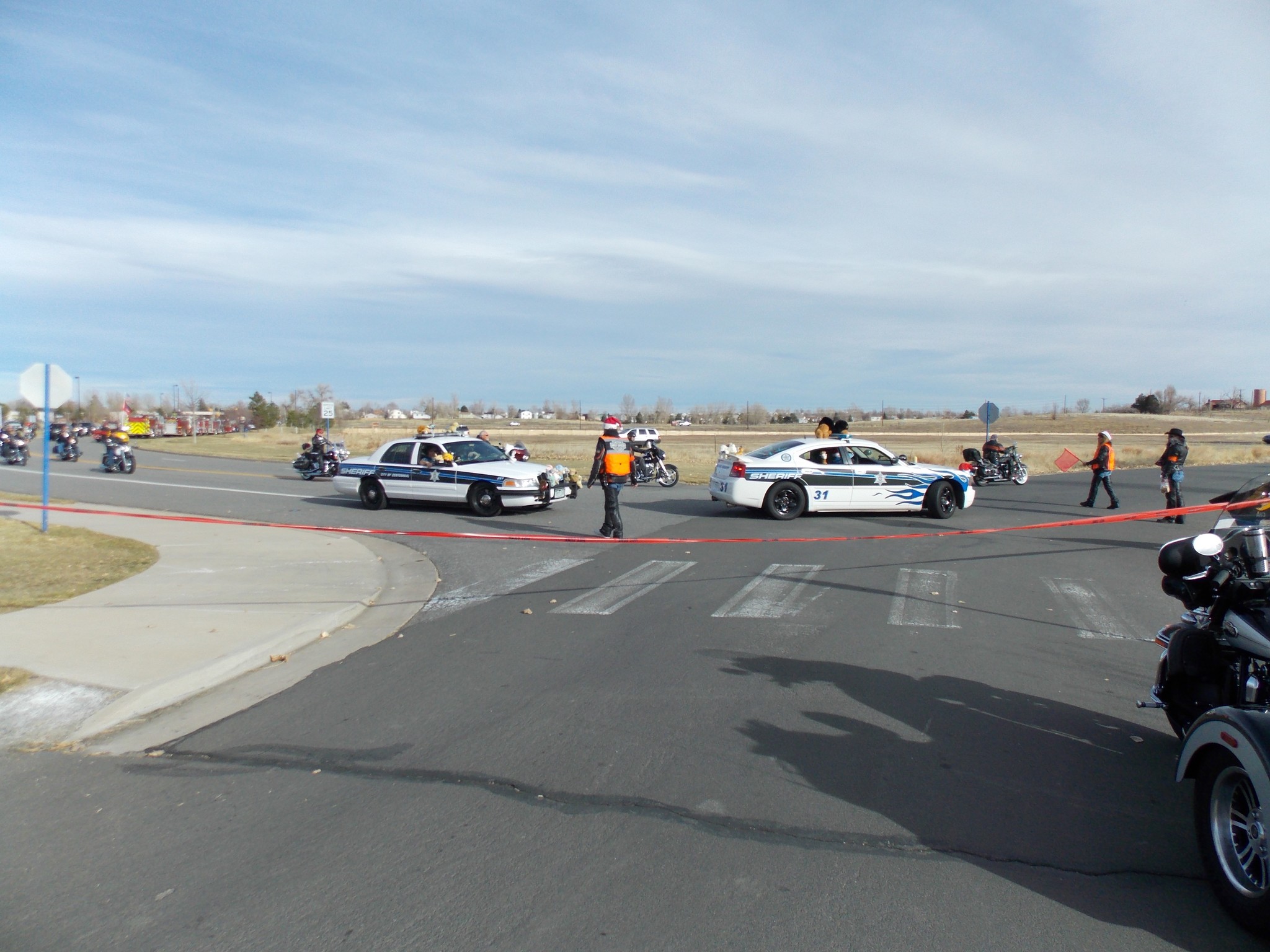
[617,427,661,445]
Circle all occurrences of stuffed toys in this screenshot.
[815,417,849,439]
[417,424,434,434]
[443,422,459,433]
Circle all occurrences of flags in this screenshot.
[124,402,133,414]
[1054,448,1080,472]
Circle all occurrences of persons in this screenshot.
[1079,432,1120,509]
[312,429,343,474]
[830,450,844,464]
[1154,428,1189,524]
[587,417,638,538]
[626,432,654,477]
[474,431,505,456]
[419,450,437,467]
[982,434,1020,480]
[819,451,828,465]
[103,419,121,468]
[4,420,83,461]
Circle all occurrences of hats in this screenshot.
[316,429,323,434]
[990,434,998,440]
[1102,431,1112,440]
[604,416,623,430]
[1164,428,1185,439]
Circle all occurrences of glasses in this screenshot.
[483,435,489,436]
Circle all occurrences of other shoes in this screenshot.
[1106,503,1120,509]
[1157,518,1168,523]
[1009,474,1017,478]
[1080,500,1093,507]
[648,474,655,477]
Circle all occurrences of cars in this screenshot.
[509,421,521,426]
[707,434,975,520]
[333,431,575,517]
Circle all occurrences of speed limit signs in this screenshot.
[321,402,335,419]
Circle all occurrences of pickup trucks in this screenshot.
[679,421,692,426]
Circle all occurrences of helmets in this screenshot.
[627,432,636,437]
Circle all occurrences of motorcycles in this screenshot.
[293,435,350,481]
[627,439,679,487]
[57,428,83,463]
[0,427,31,467]
[1135,471,1270,940]
[89,421,137,474]
[958,437,1029,486]
[491,440,530,462]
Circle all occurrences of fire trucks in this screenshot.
[120,411,240,439]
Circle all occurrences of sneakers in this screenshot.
[594,529,610,538]
